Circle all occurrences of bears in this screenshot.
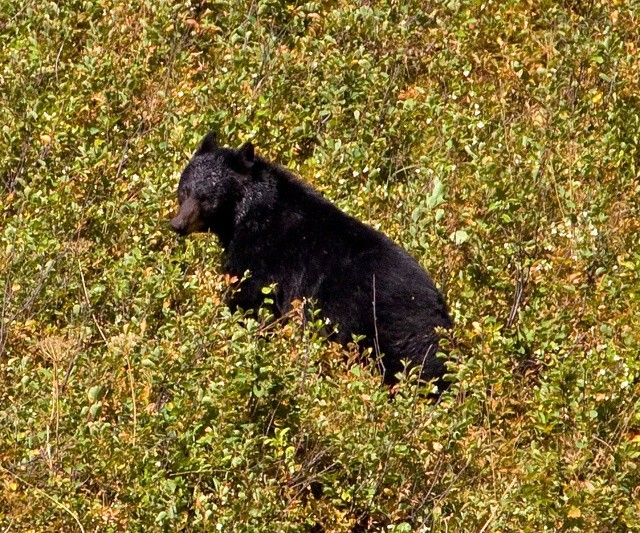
[169,130,455,405]
